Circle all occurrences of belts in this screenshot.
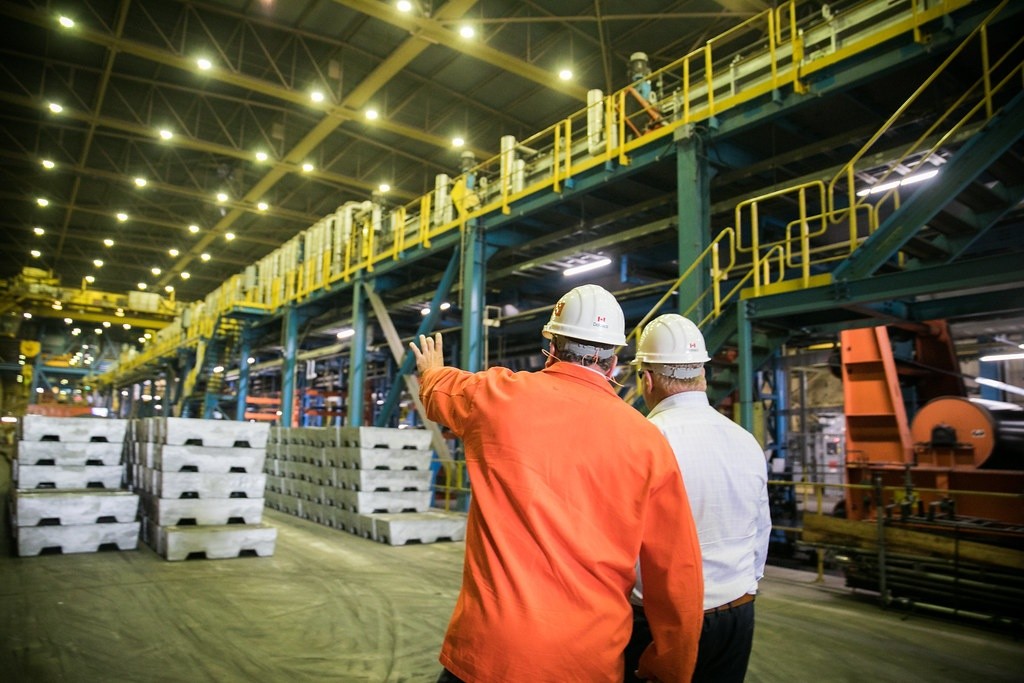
[702,594,754,614]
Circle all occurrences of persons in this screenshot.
[408,284,703,683]
[630,313,774,683]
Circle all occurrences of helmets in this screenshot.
[540,284,628,346]
[629,314,712,365]
